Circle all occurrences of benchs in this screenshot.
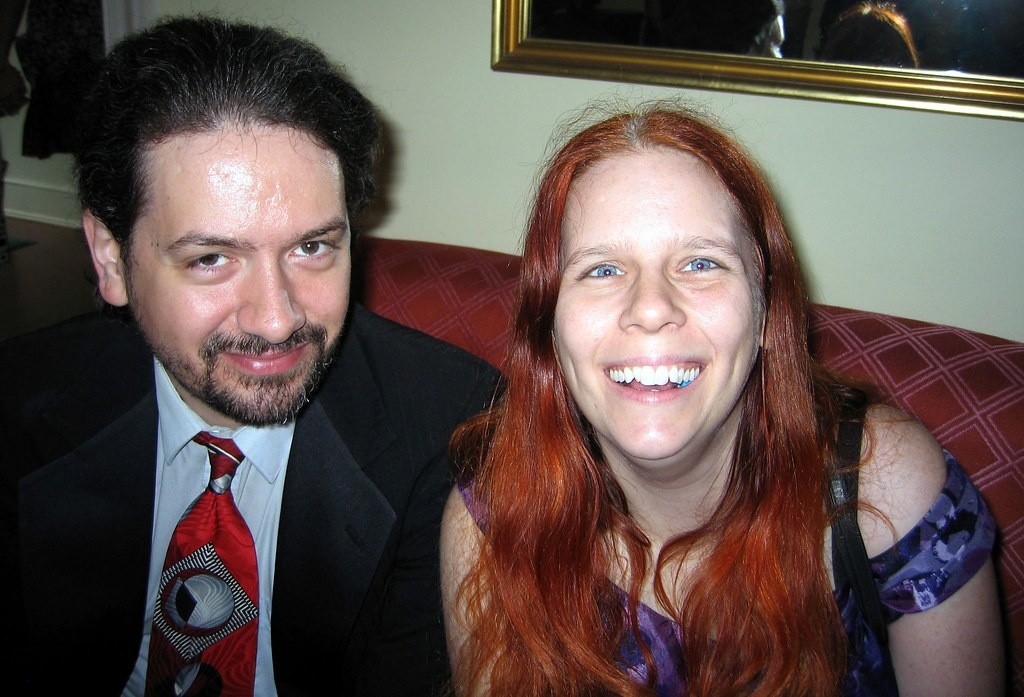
[350,236,1024,697]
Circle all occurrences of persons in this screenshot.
[688,0,786,58]
[438,109,1007,697]
[0,15,509,697]
[817,5,918,69]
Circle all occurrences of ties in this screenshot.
[143,432,259,697]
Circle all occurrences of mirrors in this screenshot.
[489,0,1024,122]
